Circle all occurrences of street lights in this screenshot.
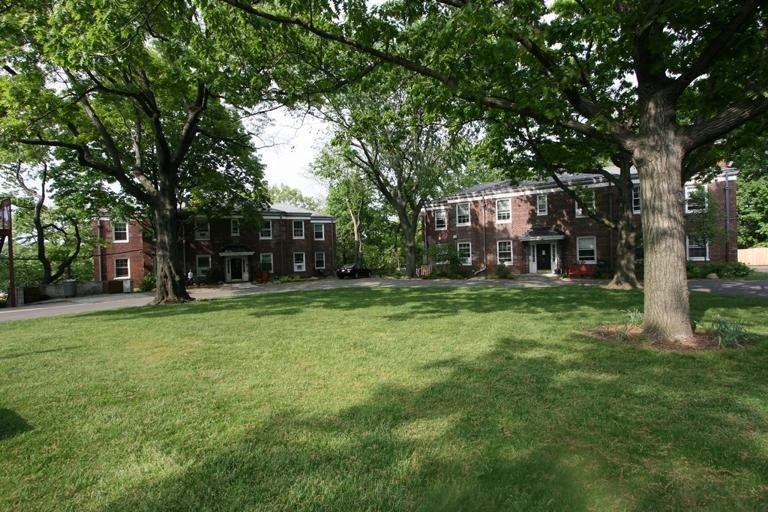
[480,190,489,281]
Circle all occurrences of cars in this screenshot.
[337,263,374,279]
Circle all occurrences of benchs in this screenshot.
[567,264,600,278]
